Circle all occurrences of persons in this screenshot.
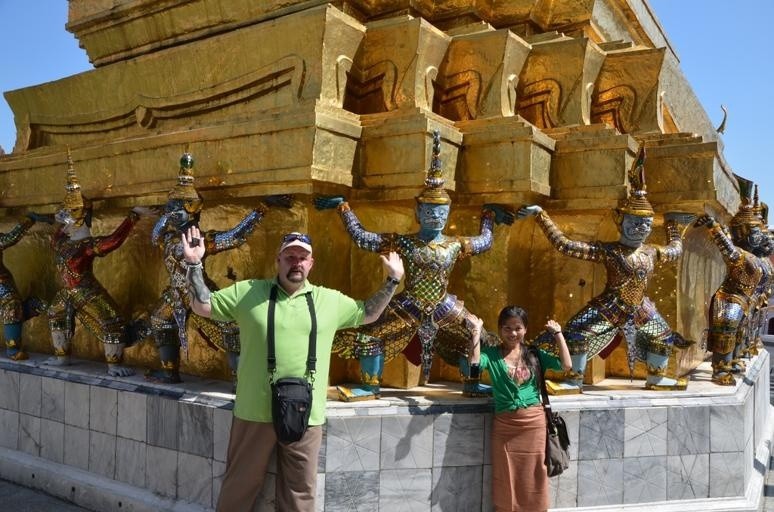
[465,303,577,511]
[692,197,772,389]
[0,209,39,362]
[145,148,263,383]
[41,144,144,375]
[514,138,699,396]
[179,221,406,512]
[312,125,515,402]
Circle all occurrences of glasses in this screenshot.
[282,233,311,244]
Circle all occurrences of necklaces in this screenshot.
[500,346,522,365]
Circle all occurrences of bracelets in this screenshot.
[183,260,201,268]
[387,274,403,288]
[472,328,481,334]
[552,329,562,340]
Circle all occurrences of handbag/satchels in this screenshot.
[271,376,314,445]
[542,410,572,477]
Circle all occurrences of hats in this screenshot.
[278,231,312,254]
